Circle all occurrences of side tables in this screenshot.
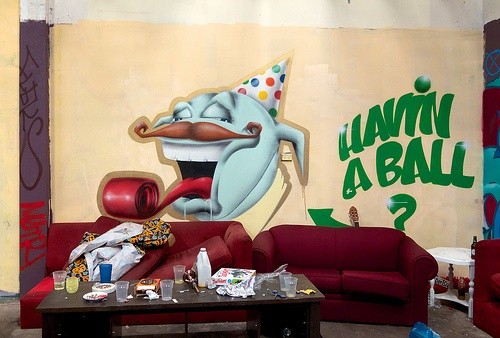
[427,247,475,319]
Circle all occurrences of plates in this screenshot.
[92,283,116,294]
[83,292,107,300]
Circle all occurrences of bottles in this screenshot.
[458,276,465,300]
[197,247,211,287]
[471,235,478,259]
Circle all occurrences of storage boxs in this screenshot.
[211,268,256,285]
[134,279,161,297]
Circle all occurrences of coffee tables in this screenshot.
[36,273,325,338]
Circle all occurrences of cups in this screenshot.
[173,265,185,284]
[284,277,298,298]
[160,280,174,301]
[52,271,66,290]
[115,281,129,303]
[278,272,291,291]
[66,277,78,293]
[99,264,112,283]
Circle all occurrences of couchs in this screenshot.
[472,239,500,338]
[19,221,253,330]
[251,225,439,327]
